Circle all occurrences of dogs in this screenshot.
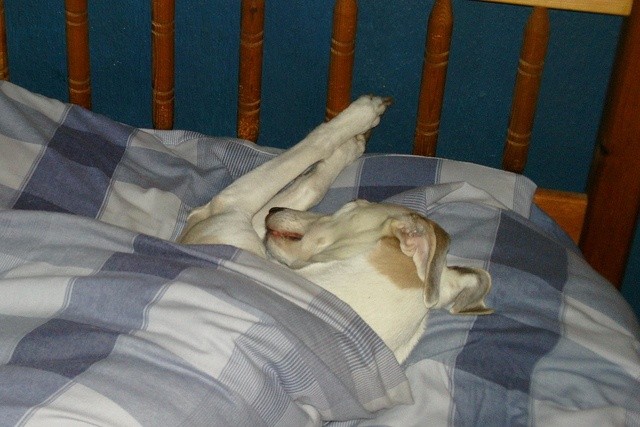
[176,94,494,369]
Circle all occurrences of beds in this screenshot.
[0,0,639,426]
